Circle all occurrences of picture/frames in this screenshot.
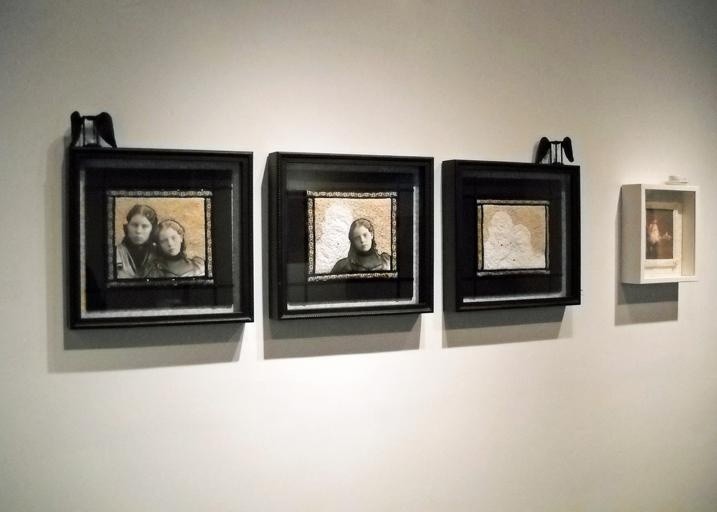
[440,159,580,313]
[62,145,256,331]
[621,183,700,285]
[266,149,438,323]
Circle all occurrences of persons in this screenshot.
[331,217,390,274]
[115,204,206,278]
[648,215,661,259]
